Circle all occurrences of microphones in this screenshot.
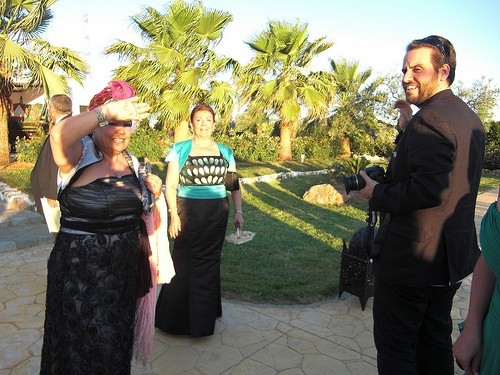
[236,222,241,239]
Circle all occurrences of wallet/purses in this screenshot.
[138,162,155,216]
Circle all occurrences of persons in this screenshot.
[39,80,163,375]
[153,105,244,338]
[452,187,500,375]
[30,95,84,240]
[349,35,485,375]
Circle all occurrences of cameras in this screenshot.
[345,165,386,195]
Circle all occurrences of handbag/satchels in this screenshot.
[225,171,240,191]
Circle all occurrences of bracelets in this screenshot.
[93,107,108,128]
[235,210,242,213]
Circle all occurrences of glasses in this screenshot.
[413,37,449,64]
